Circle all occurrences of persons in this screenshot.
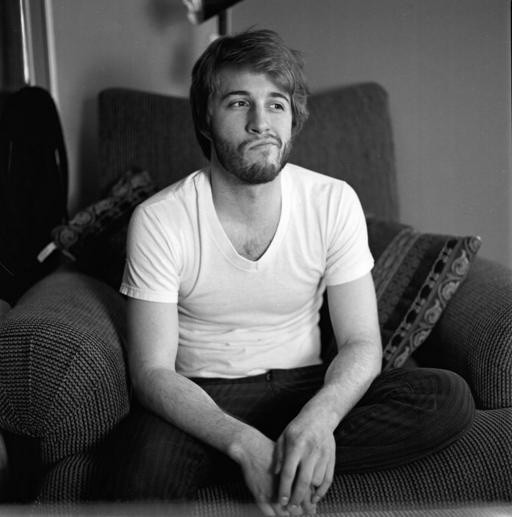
[99,28,475,517]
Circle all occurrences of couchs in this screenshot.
[0,80,511,517]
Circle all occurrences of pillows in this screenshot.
[353,216,481,371]
[50,172,158,277]
